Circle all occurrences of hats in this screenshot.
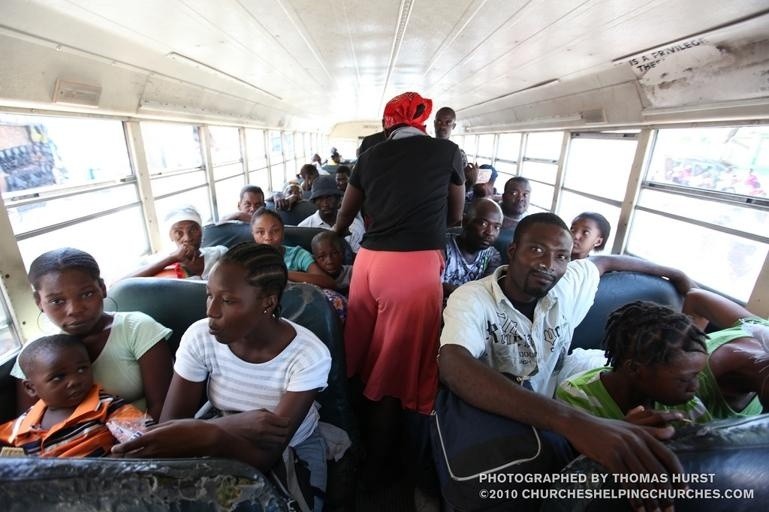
[167,207,203,241]
[309,175,344,201]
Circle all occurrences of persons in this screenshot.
[0,92,768,512]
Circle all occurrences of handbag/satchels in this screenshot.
[430,372,574,512]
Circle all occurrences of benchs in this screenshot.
[0,163,769,511]
